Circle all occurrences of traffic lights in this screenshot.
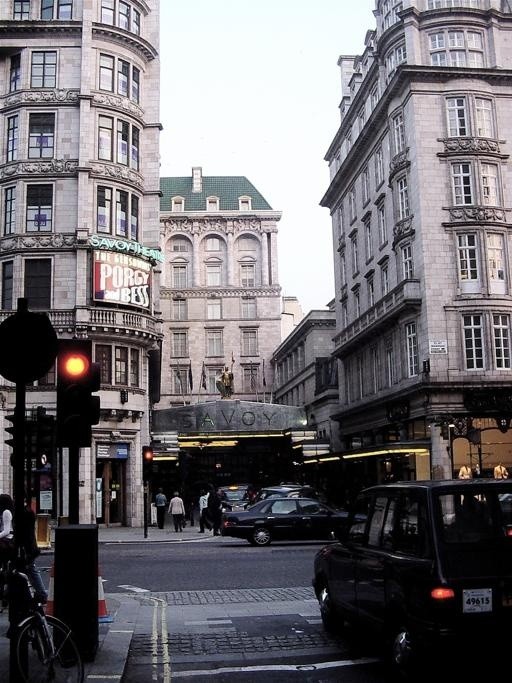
[143,444,154,482]
[57,338,94,448]
[38,406,54,450]
[5,407,16,451]
[89,361,100,426]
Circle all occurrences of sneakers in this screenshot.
[175,528,183,532]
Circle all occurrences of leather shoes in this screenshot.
[198,525,221,536]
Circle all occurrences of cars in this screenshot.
[210,480,368,543]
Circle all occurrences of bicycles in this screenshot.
[1,557,85,682]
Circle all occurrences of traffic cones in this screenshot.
[97,560,113,622]
[46,561,55,617]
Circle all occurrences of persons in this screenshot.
[154,486,167,529]
[19,494,50,603]
[197,488,212,532]
[168,490,187,530]
[1,493,17,590]
[208,485,222,536]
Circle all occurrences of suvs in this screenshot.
[312,476,512,665]
